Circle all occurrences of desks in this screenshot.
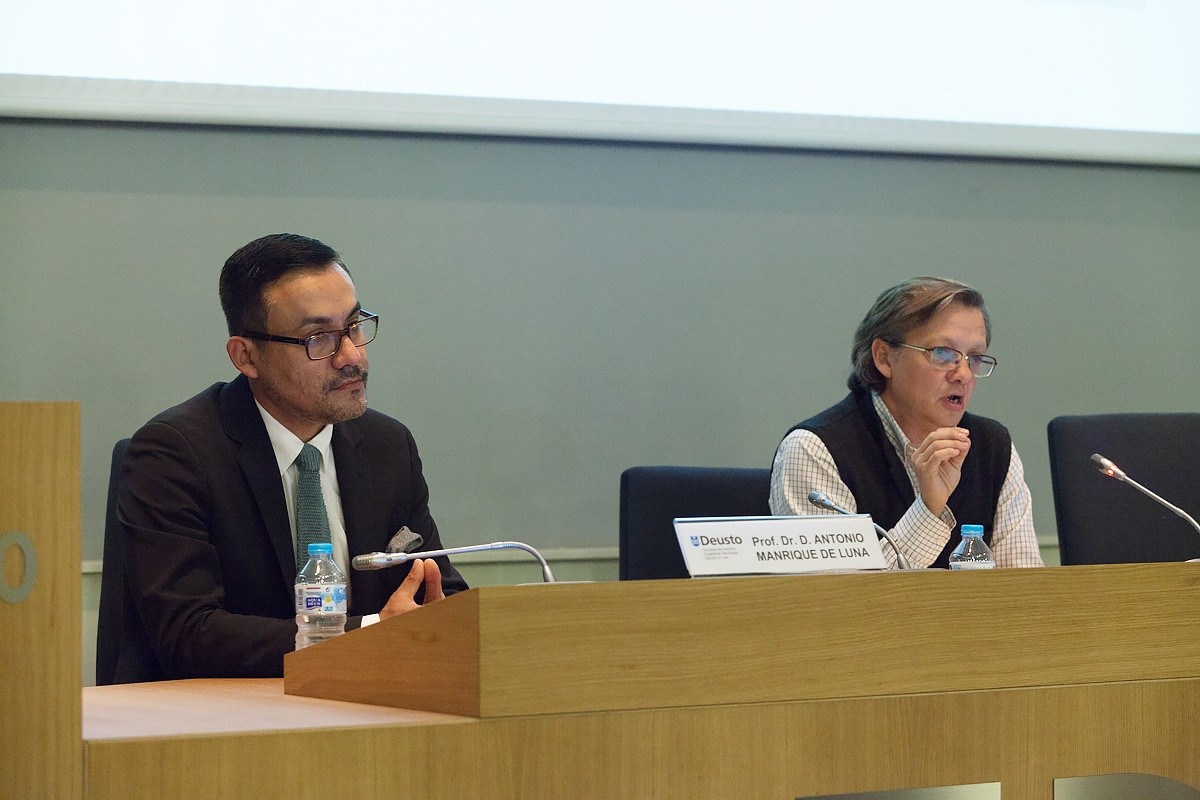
[0,401,1200,799]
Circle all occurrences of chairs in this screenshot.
[615,464,771,584]
[1046,410,1199,569]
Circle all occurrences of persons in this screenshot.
[114,233,470,678]
[770,277,1044,571]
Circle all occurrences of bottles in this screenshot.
[949,524,994,570]
[294,542,347,651]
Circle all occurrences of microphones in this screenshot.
[1088,452,1200,533]
[353,541,558,583]
[806,491,911,570]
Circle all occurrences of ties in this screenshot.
[290,448,345,570]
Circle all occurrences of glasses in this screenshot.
[898,341,998,379]
[242,308,379,361]
